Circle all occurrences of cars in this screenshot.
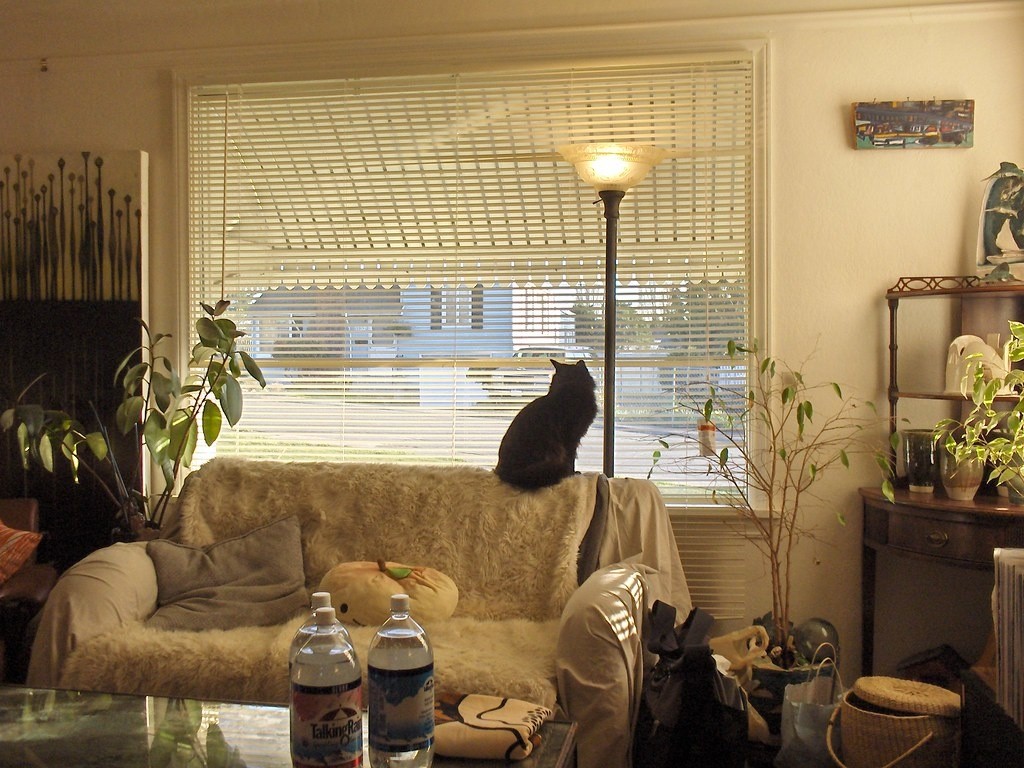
[481,342,603,401]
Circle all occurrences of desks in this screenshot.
[858,488,1024,678]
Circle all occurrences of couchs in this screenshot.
[0,498,59,686]
[25,456,692,768]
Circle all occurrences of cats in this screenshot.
[493,358,599,493]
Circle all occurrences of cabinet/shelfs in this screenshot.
[885,275,1024,491]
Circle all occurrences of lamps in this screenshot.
[554,142,668,478]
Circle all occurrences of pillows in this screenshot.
[319,561,459,627]
[146,514,312,631]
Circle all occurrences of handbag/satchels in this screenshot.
[775,645,848,768]
[632,600,748,768]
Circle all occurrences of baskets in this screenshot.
[825,676,964,767]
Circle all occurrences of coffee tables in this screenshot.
[0,685,580,768]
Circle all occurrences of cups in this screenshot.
[938,444,986,501]
[901,429,941,493]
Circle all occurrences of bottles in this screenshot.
[367,594,435,768]
[288,592,356,684]
[290,607,362,768]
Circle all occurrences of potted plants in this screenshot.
[635,338,911,763]
